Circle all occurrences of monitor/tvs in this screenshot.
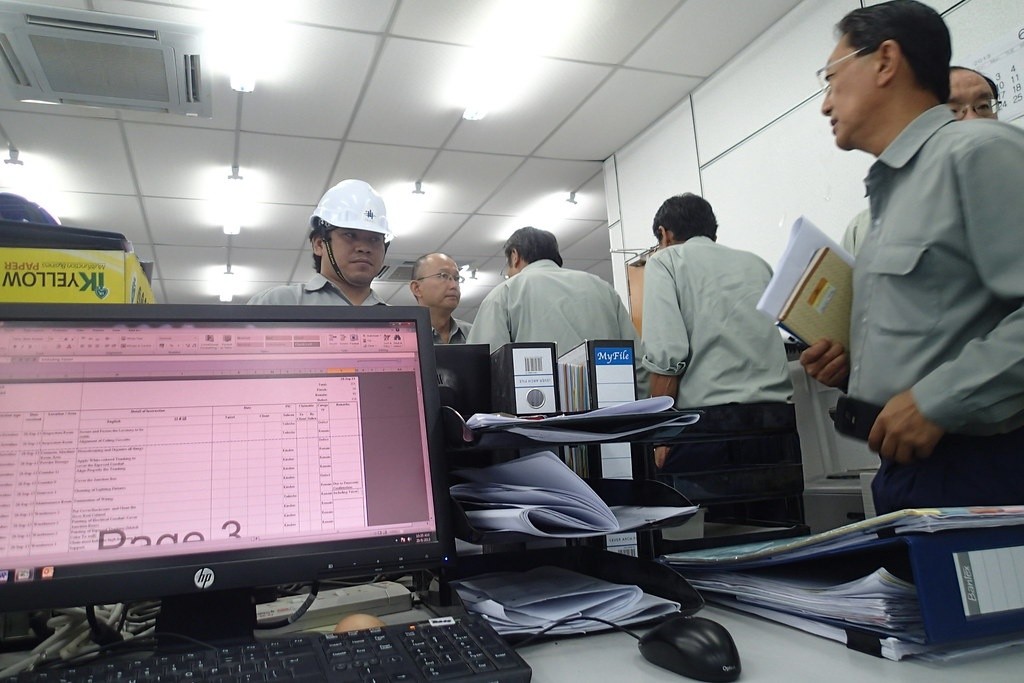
[433,344,494,471]
[0,302,455,650]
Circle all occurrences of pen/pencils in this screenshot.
[788,336,807,351]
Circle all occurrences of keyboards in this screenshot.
[0,610,532,683]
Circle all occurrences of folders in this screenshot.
[665,526,1024,647]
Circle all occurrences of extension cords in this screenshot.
[251,580,412,638]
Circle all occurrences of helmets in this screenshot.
[308,179,389,235]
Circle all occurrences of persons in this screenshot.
[799,0,1024,536]
[253,63,1000,514]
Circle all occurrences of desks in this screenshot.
[298,592,1024,683]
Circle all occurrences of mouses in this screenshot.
[638,616,742,683]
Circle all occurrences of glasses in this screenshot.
[499,258,511,280]
[947,98,1003,119]
[416,272,466,283]
[815,45,869,93]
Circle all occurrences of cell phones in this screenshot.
[833,396,885,442]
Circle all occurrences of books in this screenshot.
[781,246,856,352]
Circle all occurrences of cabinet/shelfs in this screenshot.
[804,476,865,536]
